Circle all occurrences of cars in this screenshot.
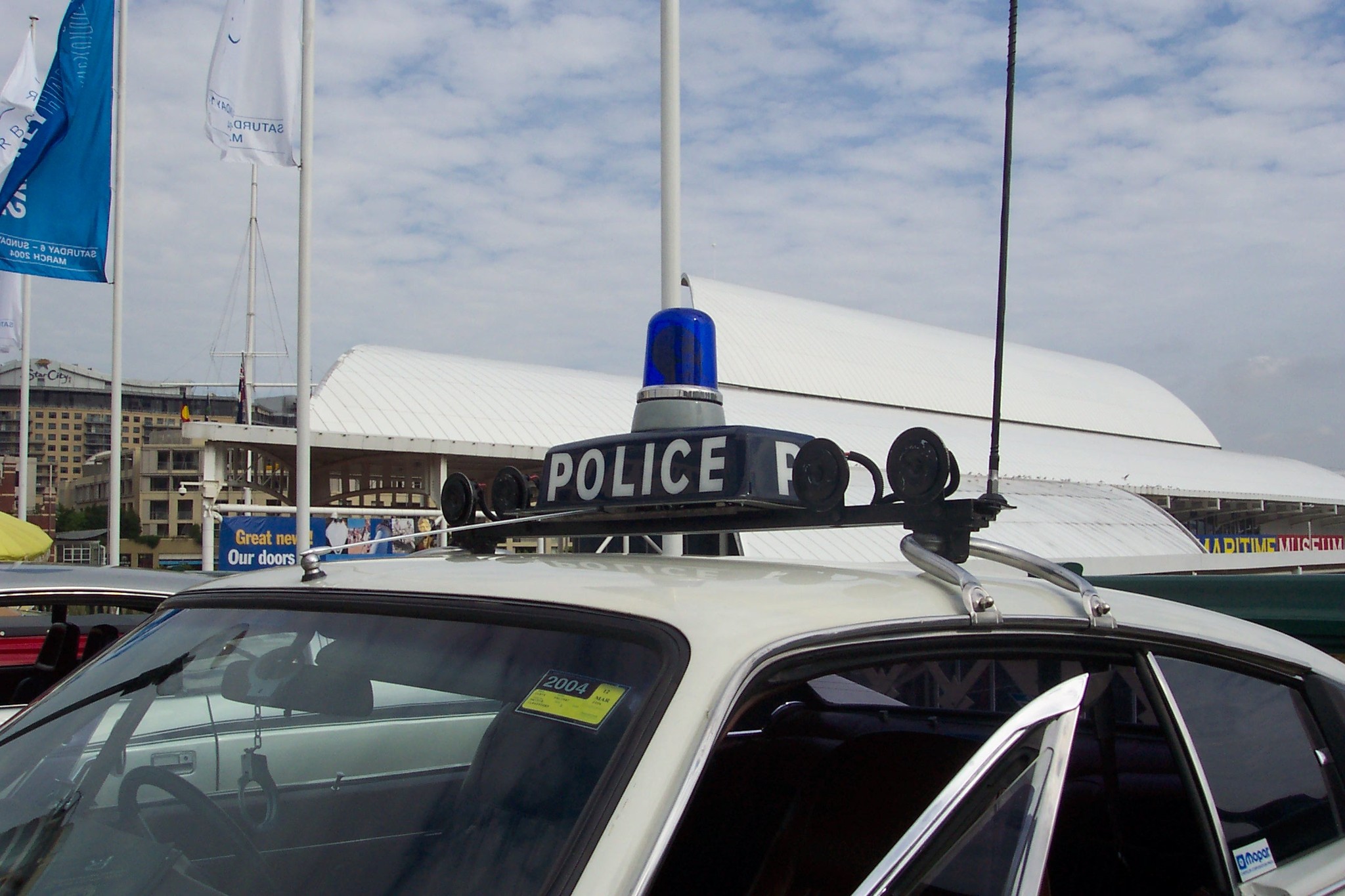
[0,303,1345,896]
[0,556,911,834]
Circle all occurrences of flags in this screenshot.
[0,25,42,192]
[0,0,115,283]
[203,0,298,168]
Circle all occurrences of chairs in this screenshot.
[8,620,80,704]
[81,622,120,666]
[746,730,1050,896]
[387,694,626,896]
